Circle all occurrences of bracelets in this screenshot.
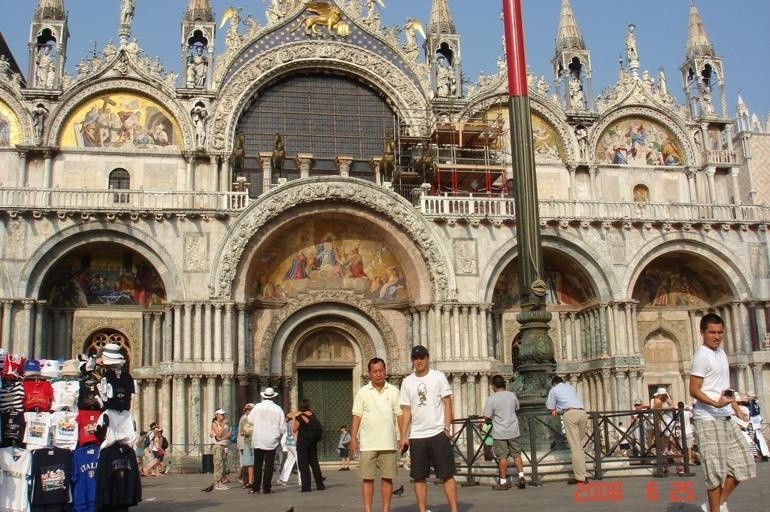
[443,425,451,432]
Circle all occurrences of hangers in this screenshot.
[0,353,134,464]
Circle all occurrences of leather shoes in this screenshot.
[242,483,274,494]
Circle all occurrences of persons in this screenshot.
[189,44,209,88]
[688,312,758,511]
[703,85,717,116]
[192,106,213,152]
[601,122,684,166]
[131,383,352,496]
[495,271,586,306]
[637,265,704,306]
[481,415,497,461]
[568,70,586,111]
[50,260,167,305]
[0,53,13,84]
[436,55,452,99]
[543,376,590,486]
[248,218,407,306]
[79,104,169,148]
[613,382,769,477]
[394,345,462,511]
[32,102,50,147]
[31,42,54,89]
[481,374,527,492]
[348,357,410,511]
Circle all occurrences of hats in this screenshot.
[260,387,278,398]
[654,387,666,396]
[411,346,428,358]
[215,408,226,415]
[102,344,125,359]
[23,359,81,378]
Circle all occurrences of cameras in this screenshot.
[723,389,735,397]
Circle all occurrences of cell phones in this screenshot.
[401,445,408,455]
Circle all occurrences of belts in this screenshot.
[565,408,584,411]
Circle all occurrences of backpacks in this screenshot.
[302,413,322,441]
[159,436,168,449]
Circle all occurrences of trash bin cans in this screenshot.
[202,454,213,474]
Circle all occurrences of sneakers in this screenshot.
[516,478,525,489]
[214,485,232,491]
[276,479,287,486]
[492,483,508,490]
[338,467,350,471]
[701,500,729,512]
